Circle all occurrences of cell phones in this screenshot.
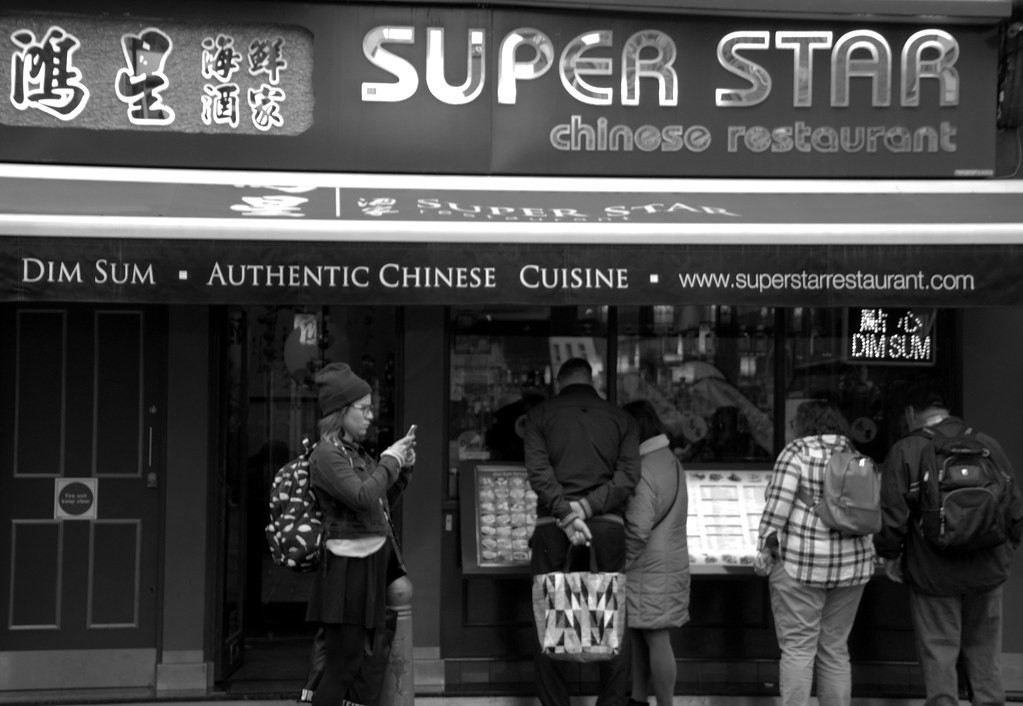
[405,425,418,437]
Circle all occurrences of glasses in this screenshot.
[351,403,375,417]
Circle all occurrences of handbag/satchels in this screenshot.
[297,608,398,706]
[531,543,627,665]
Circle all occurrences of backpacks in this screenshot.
[798,439,883,536]
[265,435,353,574]
[902,423,1012,597]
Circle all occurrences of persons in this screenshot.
[299,363,418,706]
[882,385,1021,706]
[757,398,882,705]
[619,399,692,706]
[525,356,652,706]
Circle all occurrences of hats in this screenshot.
[314,362,372,417]
